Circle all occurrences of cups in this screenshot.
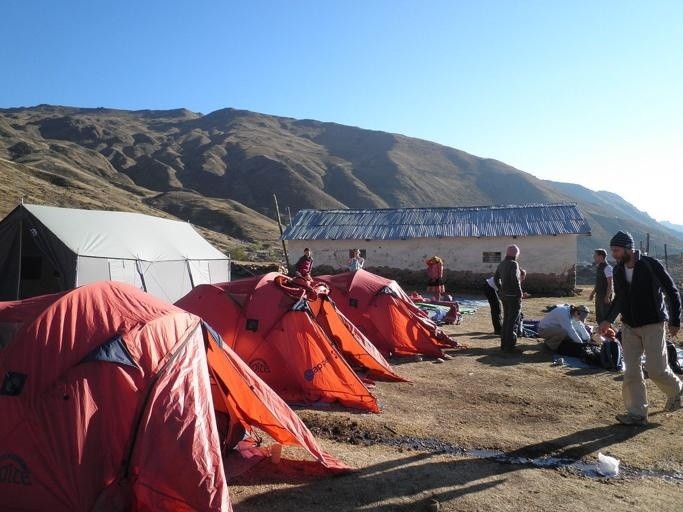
[271,444,281,464]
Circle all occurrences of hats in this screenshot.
[506,245,518,256]
[602,329,615,337]
[610,230,633,248]
[576,305,589,323]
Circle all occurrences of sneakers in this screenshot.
[614,413,648,426]
[542,343,566,354]
[664,387,682,412]
[500,344,521,353]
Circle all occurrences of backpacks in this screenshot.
[642,341,683,375]
[599,340,623,372]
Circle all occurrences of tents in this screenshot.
[172,272,414,415]
[1,282,360,512]
[0,196,231,306]
[307,270,460,361]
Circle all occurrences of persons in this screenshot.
[350,248,365,272]
[493,245,523,354]
[589,249,614,325]
[427,256,444,303]
[484,269,526,334]
[598,230,683,426]
[537,303,683,376]
[296,248,313,277]
